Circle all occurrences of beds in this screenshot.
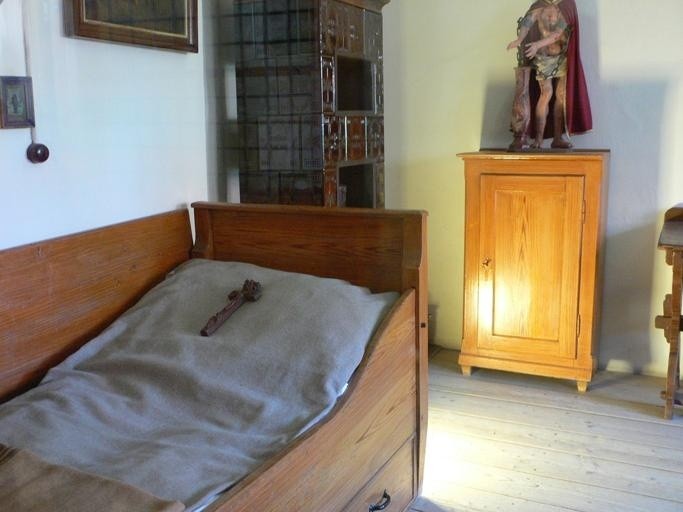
[1,195,431,512]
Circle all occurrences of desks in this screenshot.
[655,206,683,420]
[457,146,613,390]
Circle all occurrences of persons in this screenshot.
[507,0,592,148]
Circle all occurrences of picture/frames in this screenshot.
[69,0,201,52]
[0,74,36,131]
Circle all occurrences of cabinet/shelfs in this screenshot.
[235,0,387,205]
